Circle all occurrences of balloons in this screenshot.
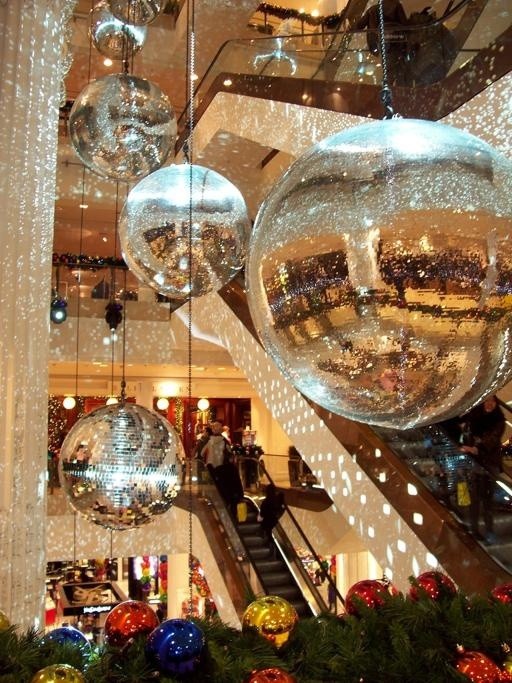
[140,555,167,622]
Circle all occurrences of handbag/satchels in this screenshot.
[237,501,247,521]
[457,482,471,507]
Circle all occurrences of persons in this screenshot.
[349,0,413,86]
[47,567,115,650]
[296,547,336,615]
[193,421,245,526]
[428,409,472,513]
[466,396,505,545]
[256,484,286,562]
[409,3,458,82]
